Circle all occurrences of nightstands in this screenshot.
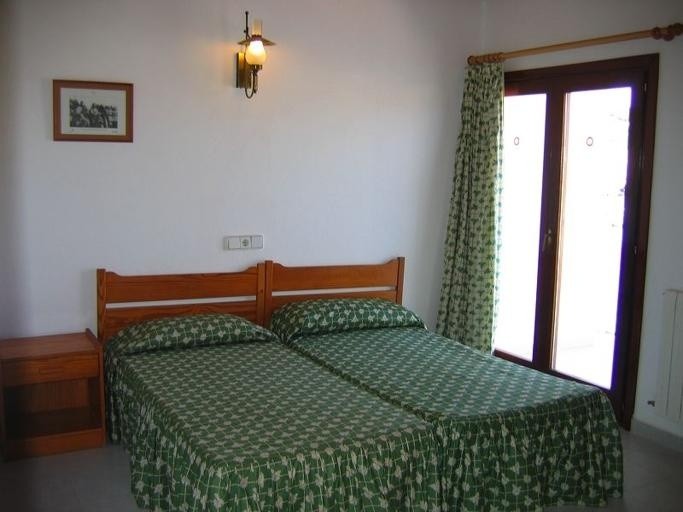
[0,327,106,463]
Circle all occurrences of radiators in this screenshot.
[647,288,683,425]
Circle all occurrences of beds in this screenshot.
[95,255,436,511]
[265,256,625,512]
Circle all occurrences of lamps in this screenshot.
[235,11,276,99]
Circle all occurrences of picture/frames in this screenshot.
[51,79,133,143]
